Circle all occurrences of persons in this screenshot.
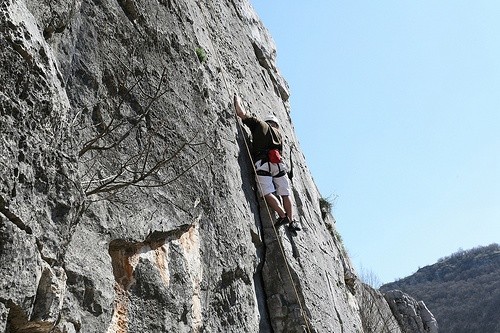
[233,91,303,232]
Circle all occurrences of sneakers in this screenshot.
[289,221,301,231]
[272,213,290,229]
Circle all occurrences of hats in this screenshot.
[264,115,279,127]
